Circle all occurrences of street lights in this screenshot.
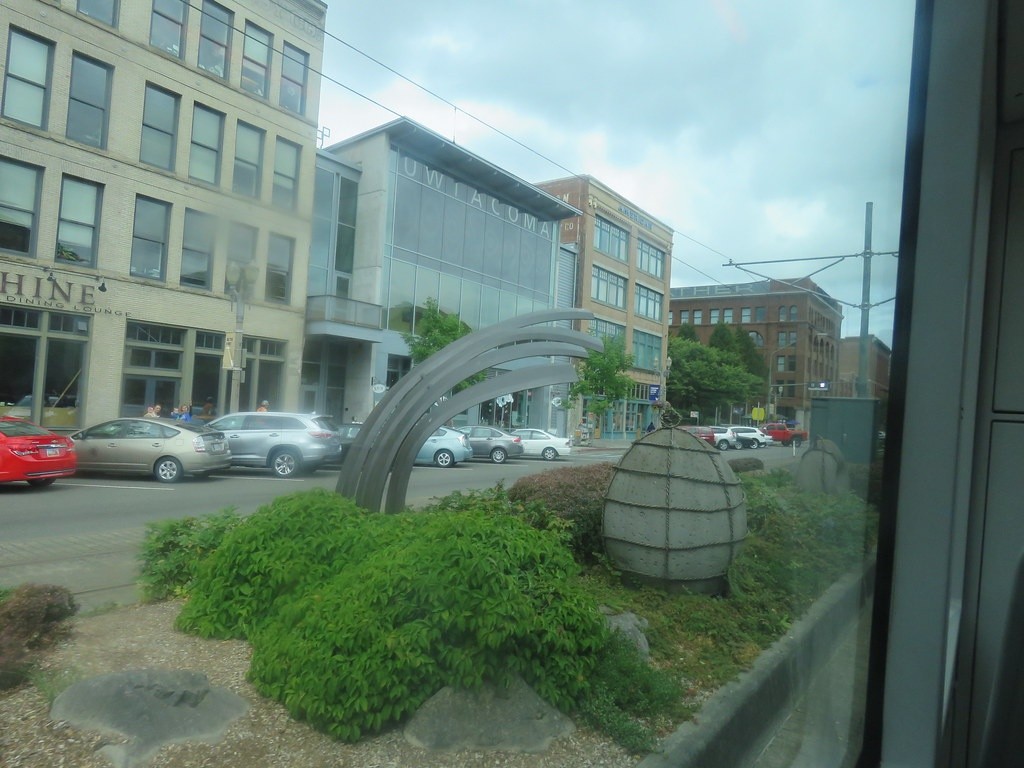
[225,256,260,411]
[766,330,830,423]
[652,357,673,430]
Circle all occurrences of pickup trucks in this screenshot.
[756,420,808,448]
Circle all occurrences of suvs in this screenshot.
[204,409,343,478]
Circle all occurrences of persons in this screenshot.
[647,422,656,433]
[142,396,269,421]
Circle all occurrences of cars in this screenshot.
[455,422,524,465]
[326,419,363,463]
[66,415,233,483]
[677,423,774,451]
[511,428,572,461]
[0,415,78,488]
[412,426,473,468]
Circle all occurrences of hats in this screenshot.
[207,397,212,401]
[261,400,269,406]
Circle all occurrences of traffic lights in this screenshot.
[808,380,827,389]
